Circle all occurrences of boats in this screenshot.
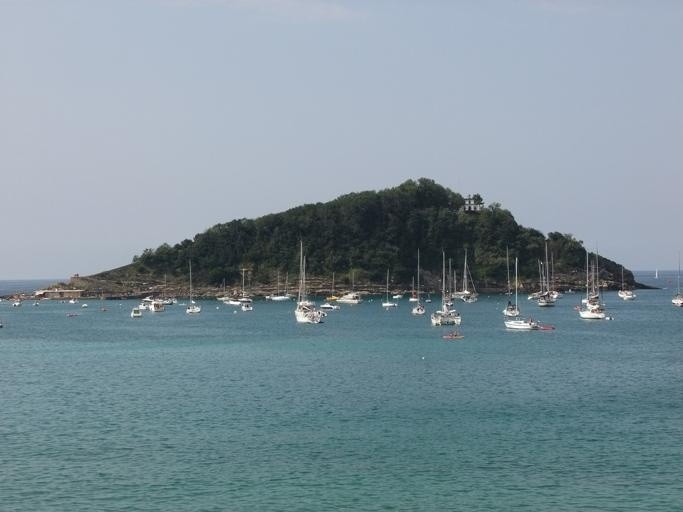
[11,288,178,320]
[442,332,463,340]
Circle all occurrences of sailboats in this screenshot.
[653,269,658,279]
[668,251,683,309]
